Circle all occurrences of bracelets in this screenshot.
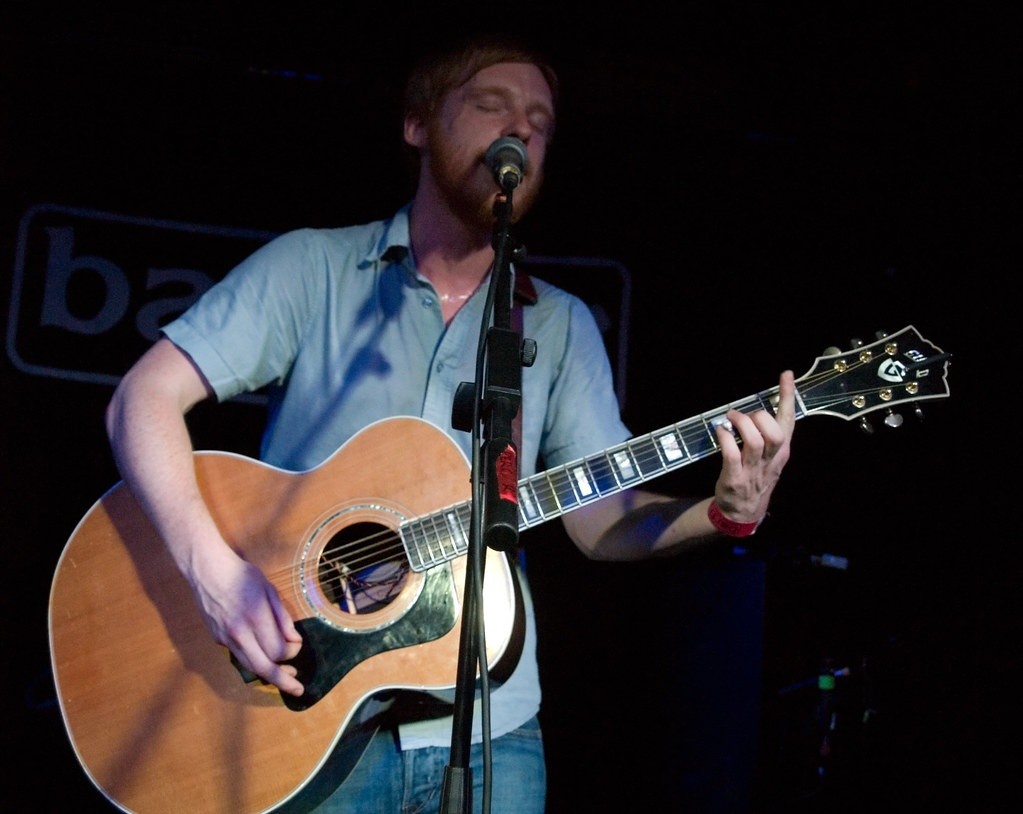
[706,496,770,537]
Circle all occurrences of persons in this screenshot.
[100,36,800,814]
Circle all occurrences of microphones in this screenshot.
[483,134,528,190]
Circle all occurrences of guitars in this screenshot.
[44,324,953,814]
